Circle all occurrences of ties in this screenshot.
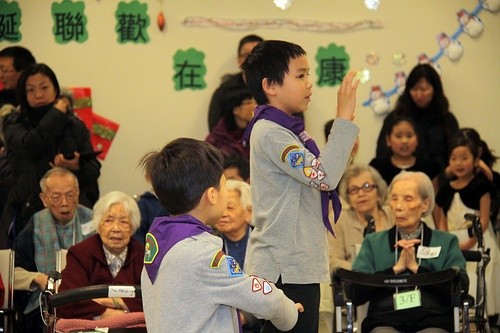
[240,105,342,240]
[143,214,213,286]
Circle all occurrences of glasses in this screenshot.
[0,65,16,73]
[347,182,377,194]
[42,189,79,202]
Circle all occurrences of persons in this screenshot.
[135,35,266,333]
[325,64,500,333]
[10,166,99,333]
[244,40,359,333]
[0,46,100,249]
[141,137,305,333]
[57,191,147,333]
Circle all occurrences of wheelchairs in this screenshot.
[330,212,494,333]
[38,271,142,333]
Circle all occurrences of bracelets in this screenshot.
[111,298,121,309]
[55,99,69,109]
